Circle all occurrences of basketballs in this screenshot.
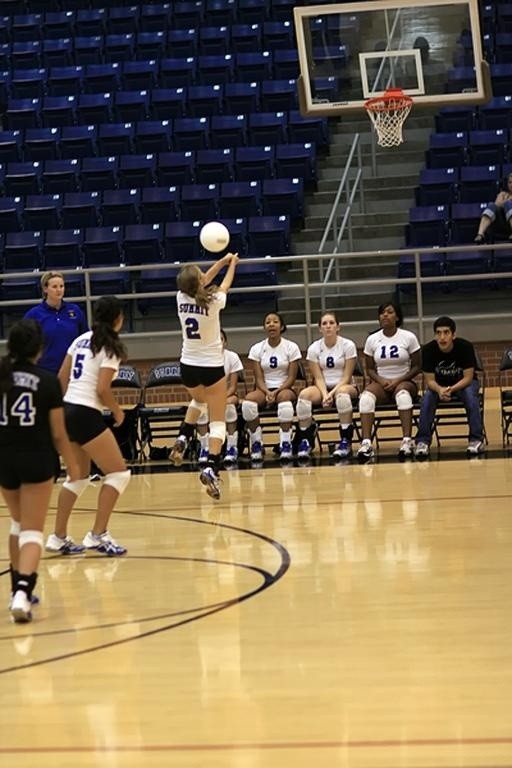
[199,222,230,253]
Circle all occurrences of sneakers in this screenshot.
[10,590,33,622]
[414,441,430,456]
[168,434,188,467]
[464,440,484,454]
[199,466,221,499]
[397,440,413,457]
[45,534,86,554]
[197,437,312,464]
[333,436,353,458]
[82,530,127,554]
[356,442,375,457]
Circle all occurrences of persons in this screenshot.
[53,296,131,556]
[243,312,302,464]
[194,328,244,463]
[298,311,358,462]
[414,315,486,455]
[305,28,475,105]
[0,315,79,623]
[359,302,424,460]
[169,250,240,499]
[473,174,512,243]
[45,548,147,767]
[22,270,93,484]
